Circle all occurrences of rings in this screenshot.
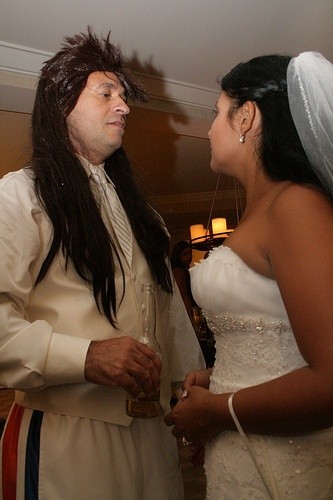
[179,436,191,449]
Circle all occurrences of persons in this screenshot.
[0,23,208,500]
[166,49,333,500]
[171,242,202,344]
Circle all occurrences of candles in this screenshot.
[190,218,234,244]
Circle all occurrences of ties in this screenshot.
[95,166,132,272]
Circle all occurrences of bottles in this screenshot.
[169,380,206,500]
[126,284,164,419]
